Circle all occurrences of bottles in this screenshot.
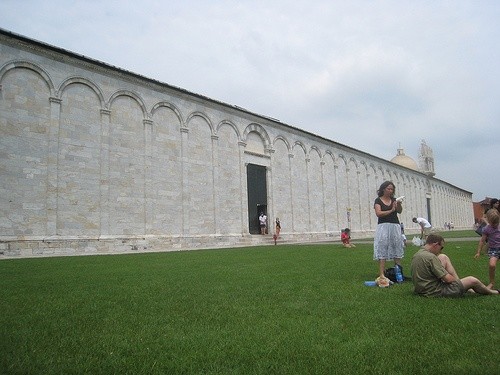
[394,262,403,283]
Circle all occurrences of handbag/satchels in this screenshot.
[475,222,485,236]
[384,267,405,283]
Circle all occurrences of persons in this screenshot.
[473,198,500,244]
[373,181,404,283]
[341,228,355,248]
[444,222,455,231]
[412,217,431,246]
[472,208,500,288]
[259,212,267,235]
[411,234,500,296]
[275,217,282,237]
[401,222,407,247]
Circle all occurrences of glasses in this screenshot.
[439,244,444,250]
